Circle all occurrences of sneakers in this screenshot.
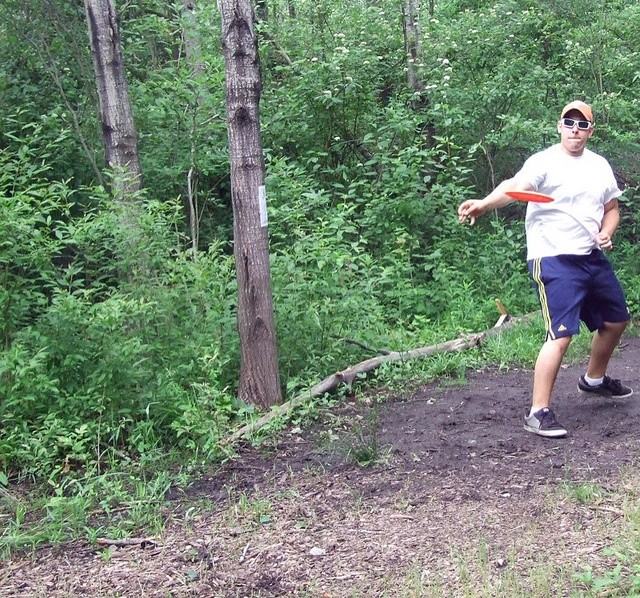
[524,407,567,438]
[576,376,633,398]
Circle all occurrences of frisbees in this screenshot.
[506,190,554,204]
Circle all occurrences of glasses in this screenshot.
[562,117,596,130]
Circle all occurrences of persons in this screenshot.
[457,99,633,436]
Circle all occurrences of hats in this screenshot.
[561,99,593,121]
